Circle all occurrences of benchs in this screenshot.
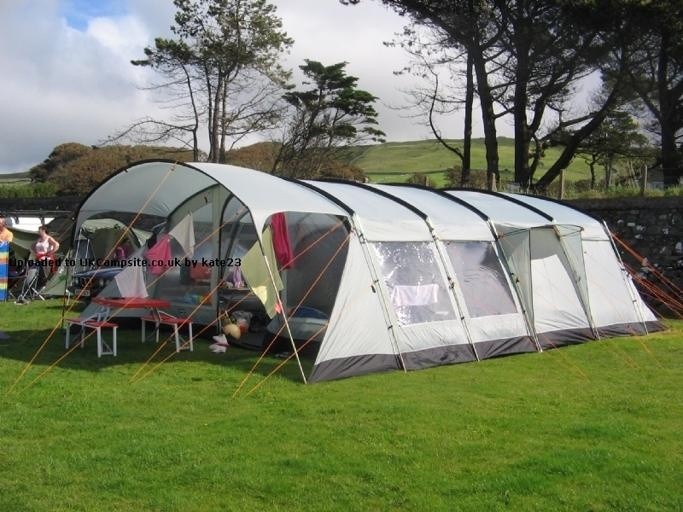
[141,315,193,354]
[64,318,119,357]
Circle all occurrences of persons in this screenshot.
[27,224,61,286]
[0,217,13,244]
[115,237,130,260]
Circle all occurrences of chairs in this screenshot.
[9,261,45,303]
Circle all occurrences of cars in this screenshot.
[66,265,125,306]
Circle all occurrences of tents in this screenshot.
[69,154,672,388]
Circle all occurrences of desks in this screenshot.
[93,298,171,324]
